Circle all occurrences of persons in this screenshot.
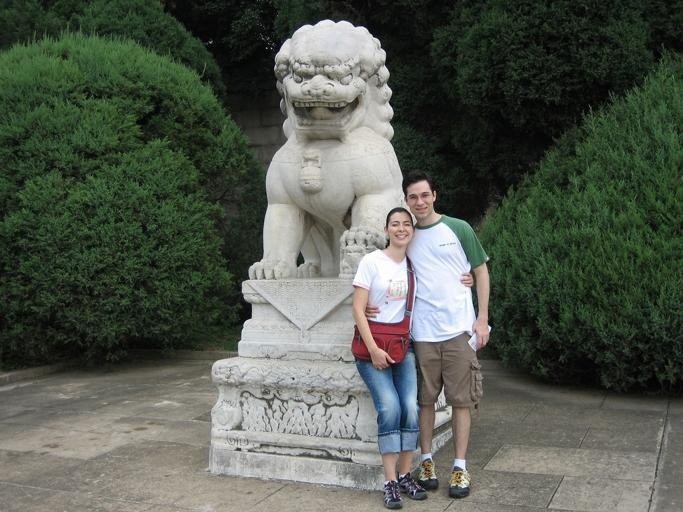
[365,170,491,499]
[351,208,474,510]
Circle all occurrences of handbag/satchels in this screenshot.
[352,311,410,364]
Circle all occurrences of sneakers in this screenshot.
[398,472,427,500]
[383,481,403,508]
[415,459,438,490]
[448,466,471,498]
